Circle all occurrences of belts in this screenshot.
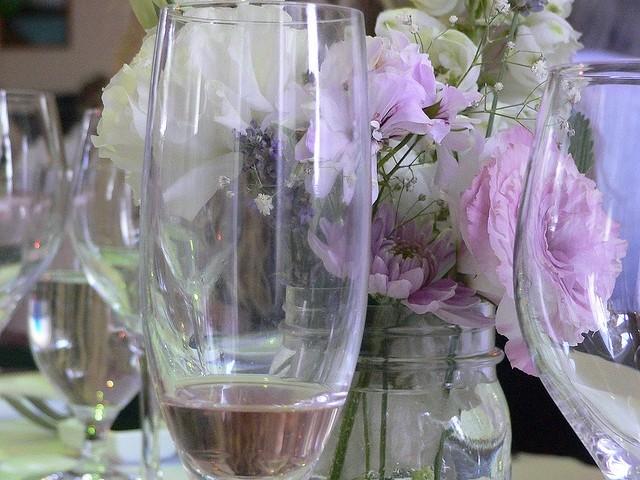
[68,108,168,479]
[26,168,138,479]
[139,2,370,479]
[512,61,640,480]
[1,91,73,334]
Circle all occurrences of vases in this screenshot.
[87,0,619,480]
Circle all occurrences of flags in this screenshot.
[264,286,516,480]
